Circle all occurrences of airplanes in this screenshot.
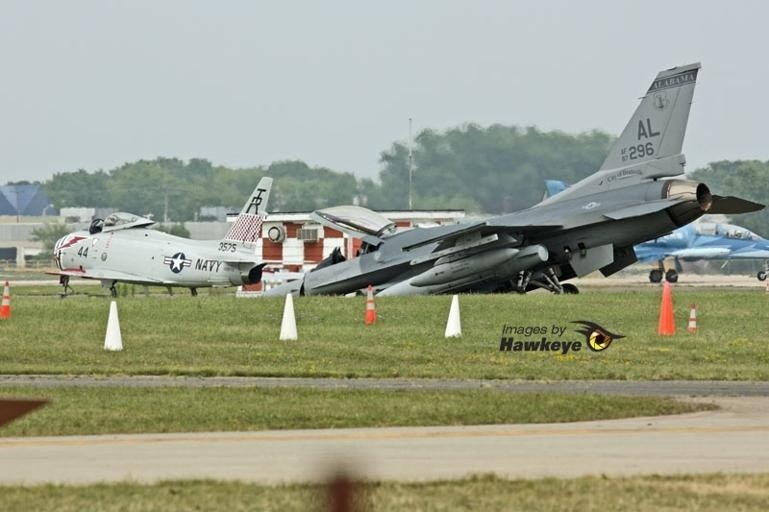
[40,177,316,298]
[543,180,769,283]
[257,61,765,297]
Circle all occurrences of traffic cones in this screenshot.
[686,303,698,336]
[1,281,12,322]
[365,284,377,325]
[280,292,298,341]
[657,281,678,338]
[443,293,462,341]
[104,299,124,351]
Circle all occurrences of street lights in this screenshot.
[42,204,54,268]
